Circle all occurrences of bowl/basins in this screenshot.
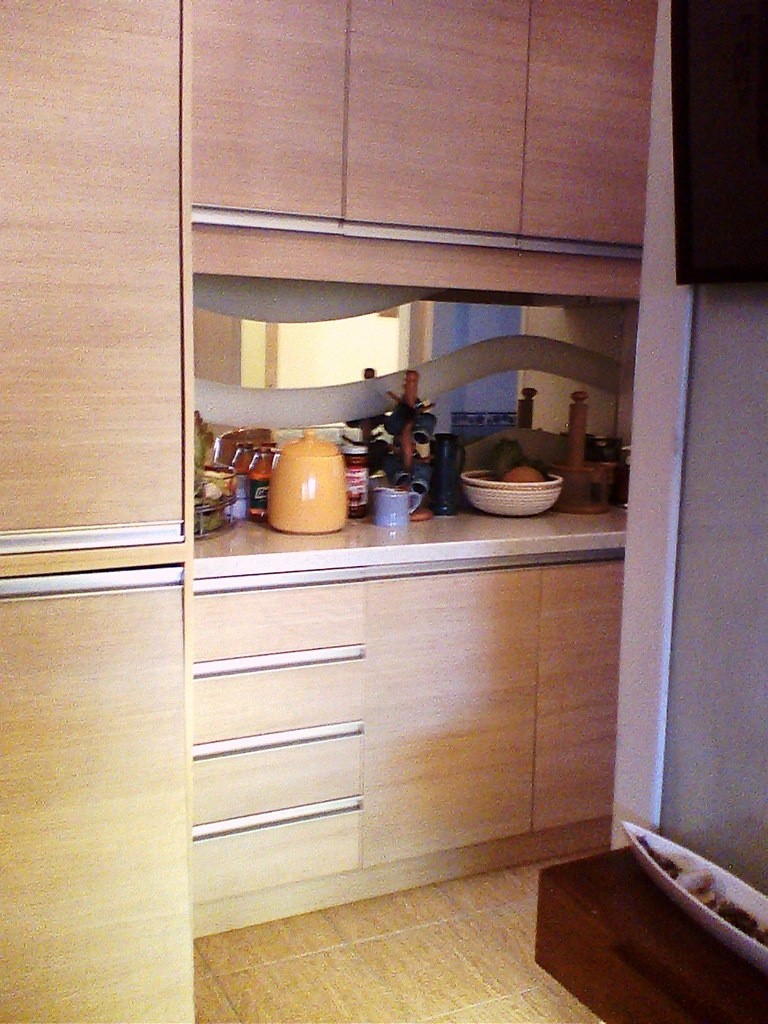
[459,470,562,516]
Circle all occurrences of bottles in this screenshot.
[430,433,458,516]
[342,446,369,518]
[247,447,273,522]
[262,442,277,461]
[231,443,255,518]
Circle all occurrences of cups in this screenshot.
[412,464,432,495]
[385,406,413,434]
[414,413,437,443]
[373,490,421,527]
[385,460,409,487]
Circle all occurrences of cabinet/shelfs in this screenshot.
[362,557,624,877]
[191,0,662,300]
[192,566,370,907]
[0,0,196,1024]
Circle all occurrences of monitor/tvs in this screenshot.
[670,0,768,286]
